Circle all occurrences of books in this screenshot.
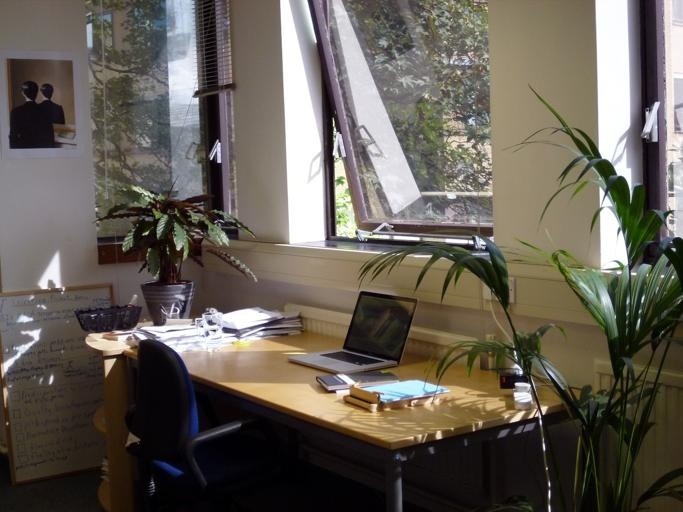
[127,305,304,351]
[315,370,451,411]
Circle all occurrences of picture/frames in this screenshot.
[0,50,84,162]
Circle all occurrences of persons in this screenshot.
[9,80,55,148]
[38,84,65,147]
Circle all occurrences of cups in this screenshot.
[195,311,223,340]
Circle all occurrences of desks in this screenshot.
[85,328,582,512]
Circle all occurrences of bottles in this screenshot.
[479,334,497,370]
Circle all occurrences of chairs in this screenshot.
[125,339,263,512]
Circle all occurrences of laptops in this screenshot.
[287,290,419,377]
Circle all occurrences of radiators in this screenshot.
[592,358,683,512]
[284,303,498,512]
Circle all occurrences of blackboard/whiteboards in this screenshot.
[0,283,118,485]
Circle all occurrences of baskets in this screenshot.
[74,304,142,333]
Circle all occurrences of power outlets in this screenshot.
[482,277,516,303]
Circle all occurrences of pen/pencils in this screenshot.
[122,294,138,329]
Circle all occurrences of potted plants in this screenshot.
[93,175,259,326]
